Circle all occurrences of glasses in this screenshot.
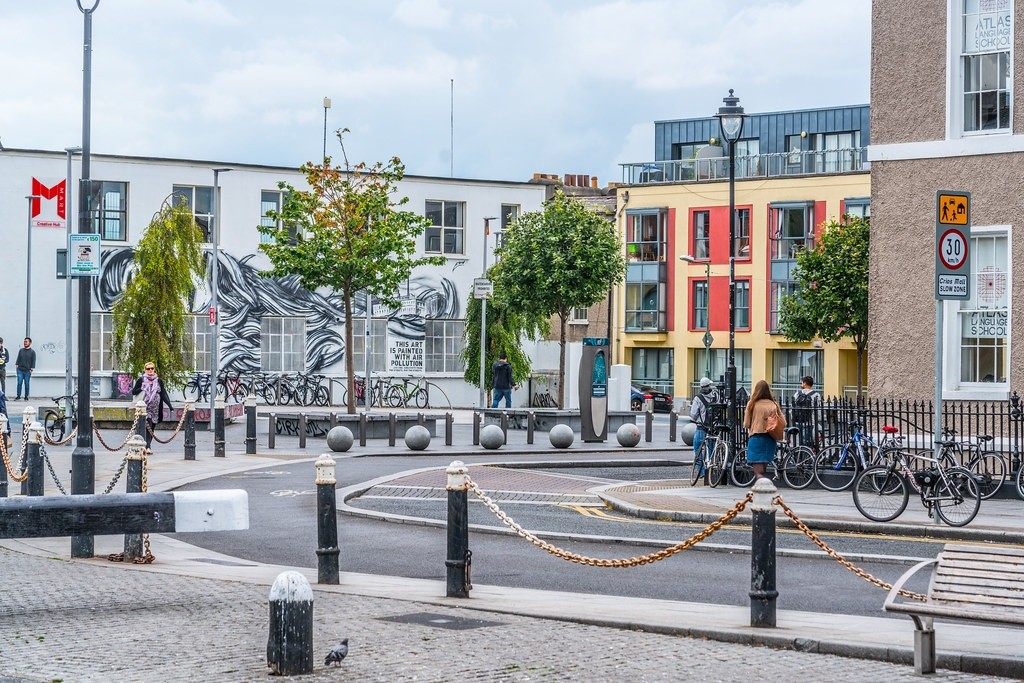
[146,367,154,370]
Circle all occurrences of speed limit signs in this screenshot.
[939,229,968,270]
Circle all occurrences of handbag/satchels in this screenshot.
[765,401,785,440]
[812,423,823,445]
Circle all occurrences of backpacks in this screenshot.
[792,390,817,423]
[690,390,721,435]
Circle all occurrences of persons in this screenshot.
[131,361,173,455]
[492,353,519,408]
[0,338,9,402]
[14,337,36,401]
[691,377,719,477]
[791,376,822,442]
[742,381,780,479]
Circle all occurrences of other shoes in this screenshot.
[15,397,21,400]
[24,398,29,401]
[147,451,153,455]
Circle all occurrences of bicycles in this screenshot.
[852,436,981,527]
[265,372,330,406]
[907,427,1007,501]
[373,371,401,408]
[44,390,79,442]
[219,370,249,404]
[184,372,228,403]
[390,375,428,409]
[732,427,818,490]
[690,420,731,488]
[1015,463,1024,501]
[813,421,910,495]
[243,369,277,406]
[343,373,375,407]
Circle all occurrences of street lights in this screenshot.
[712,89,750,455]
[208,168,233,432]
[480,217,497,410]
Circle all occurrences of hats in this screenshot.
[700,377,713,387]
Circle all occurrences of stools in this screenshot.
[642,251,656,261]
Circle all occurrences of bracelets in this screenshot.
[31,370,33,371]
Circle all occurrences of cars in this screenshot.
[631,381,672,413]
